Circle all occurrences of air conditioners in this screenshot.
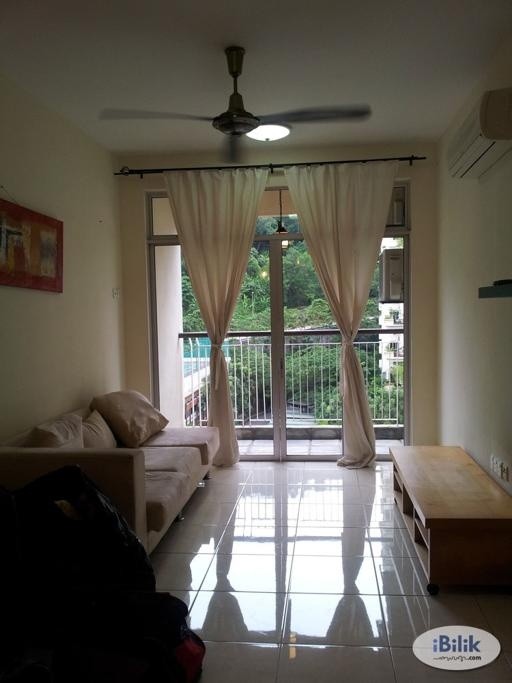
[378,250,404,303]
[447,88,512,179]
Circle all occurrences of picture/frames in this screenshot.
[0,198,63,293]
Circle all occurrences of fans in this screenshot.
[99,45,371,164]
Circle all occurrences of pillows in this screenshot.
[35,390,169,449]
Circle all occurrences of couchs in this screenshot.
[0,408,220,558]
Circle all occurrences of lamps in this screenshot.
[246,123,292,142]
[276,190,288,249]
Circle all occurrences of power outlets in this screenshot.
[490,456,508,480]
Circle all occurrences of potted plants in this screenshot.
[384,314,394,326]
[384,346,397,359]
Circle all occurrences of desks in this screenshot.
[389,446,512,596]
